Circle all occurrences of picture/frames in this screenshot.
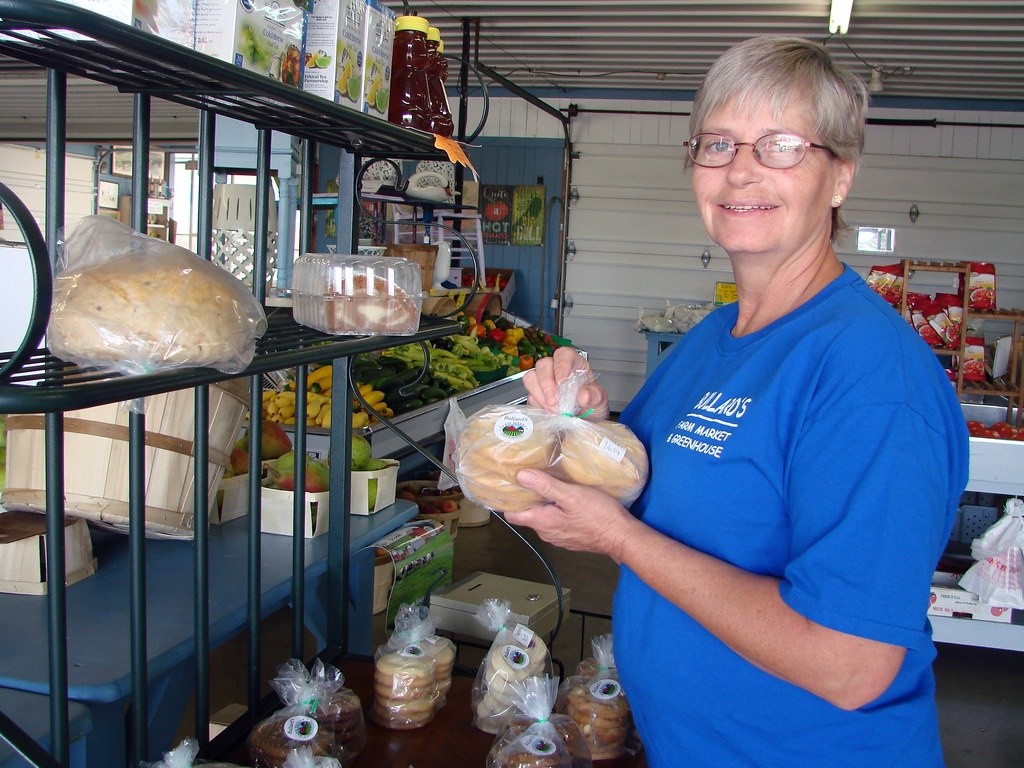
[111,144,165,184]
[100,178,120,209]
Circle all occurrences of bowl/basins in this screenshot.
[0,512,98,597]
[463,293,503,324]
[373,553,394,614]
[326,245,387,257]
[422,296,458,322]
[416,502,462,547]
[385,243,439,292]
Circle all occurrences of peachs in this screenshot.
[399,484,459,515]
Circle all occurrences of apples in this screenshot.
[218,419,388,531]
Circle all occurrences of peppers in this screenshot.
[382,313,565,391]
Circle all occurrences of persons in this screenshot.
[504,38,970,768]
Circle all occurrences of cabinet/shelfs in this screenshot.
[893,260,1024,446]
[0,1,637,767]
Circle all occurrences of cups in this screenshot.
[269,57,281,80]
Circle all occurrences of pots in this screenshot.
[398,480,465,502]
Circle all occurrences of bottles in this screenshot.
[158,175,162,196]
[389,16,432,133]
[427,27,454,139]
[150,174,155,195]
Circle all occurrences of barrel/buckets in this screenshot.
[0,374,251,539]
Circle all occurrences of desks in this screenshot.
[0,493,417,768]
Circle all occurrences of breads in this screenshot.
[251,637,629,768]
[45,252,256,364]
[458,407,648,513]
[325,273,419,335]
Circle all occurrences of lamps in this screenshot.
[829,0,854,34]
[871,67,886,90]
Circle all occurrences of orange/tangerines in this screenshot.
[306,53,389,112]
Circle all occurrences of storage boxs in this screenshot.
[431,571,570,643]
[928,587,1012,624]
[456,268,516,315]
[56,2,395,123]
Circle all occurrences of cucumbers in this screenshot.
[317,355,458,413]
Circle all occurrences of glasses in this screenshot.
[683,133,841,168]
